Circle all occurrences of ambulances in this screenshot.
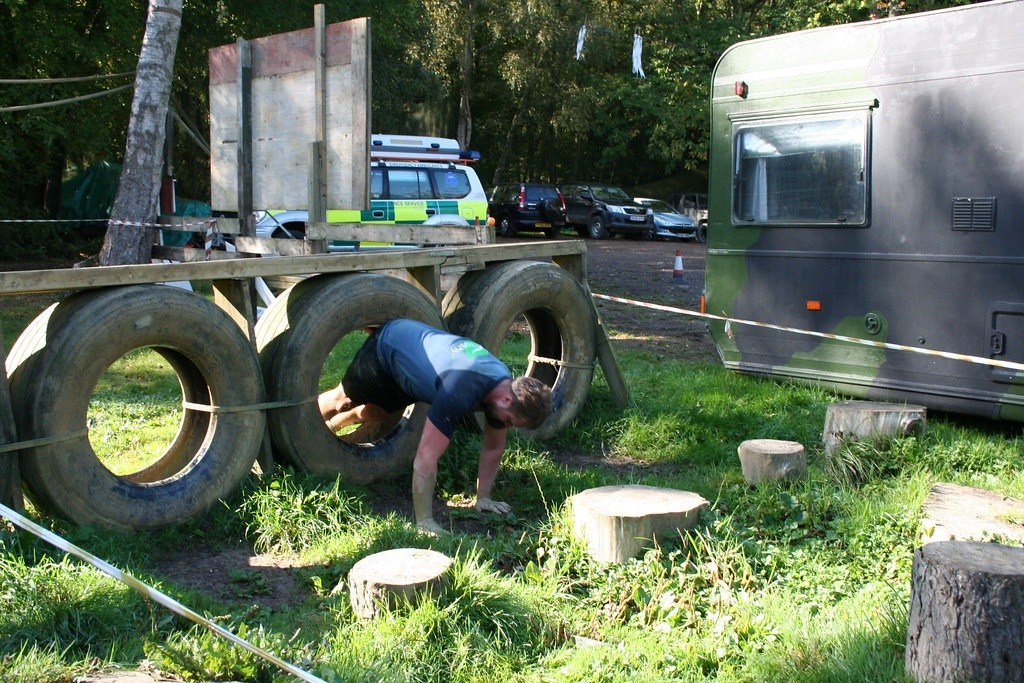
[227,133,497,252]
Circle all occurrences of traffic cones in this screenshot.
[668,249,688,284]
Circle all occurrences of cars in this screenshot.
[633,197,697,243]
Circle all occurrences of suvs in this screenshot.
[665,192,709,244]
[483,181,567,239]
[558,181,654,241]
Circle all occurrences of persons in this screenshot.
[317,319,554,539]
